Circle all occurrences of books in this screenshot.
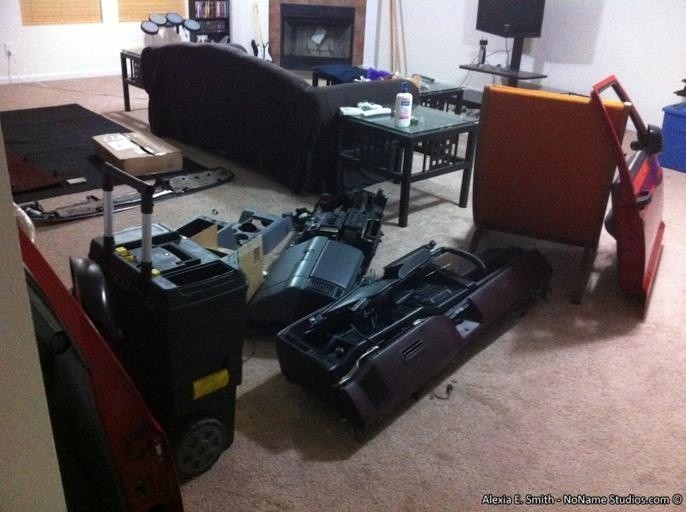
[195,1,227,19]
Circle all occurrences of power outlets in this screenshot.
[4,40,14,56]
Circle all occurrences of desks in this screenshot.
[337,102,479,226]
[120,46,148,111]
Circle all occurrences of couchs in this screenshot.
[132,40,415,199]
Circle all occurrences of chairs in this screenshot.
[472,77,634,306]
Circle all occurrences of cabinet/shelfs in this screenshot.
[187,0,230,45]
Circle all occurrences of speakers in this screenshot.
[475,0,546,39]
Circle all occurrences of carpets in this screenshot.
[0,102,210,205]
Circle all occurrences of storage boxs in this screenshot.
[658,102,686,173]
[91,129,184,175]
